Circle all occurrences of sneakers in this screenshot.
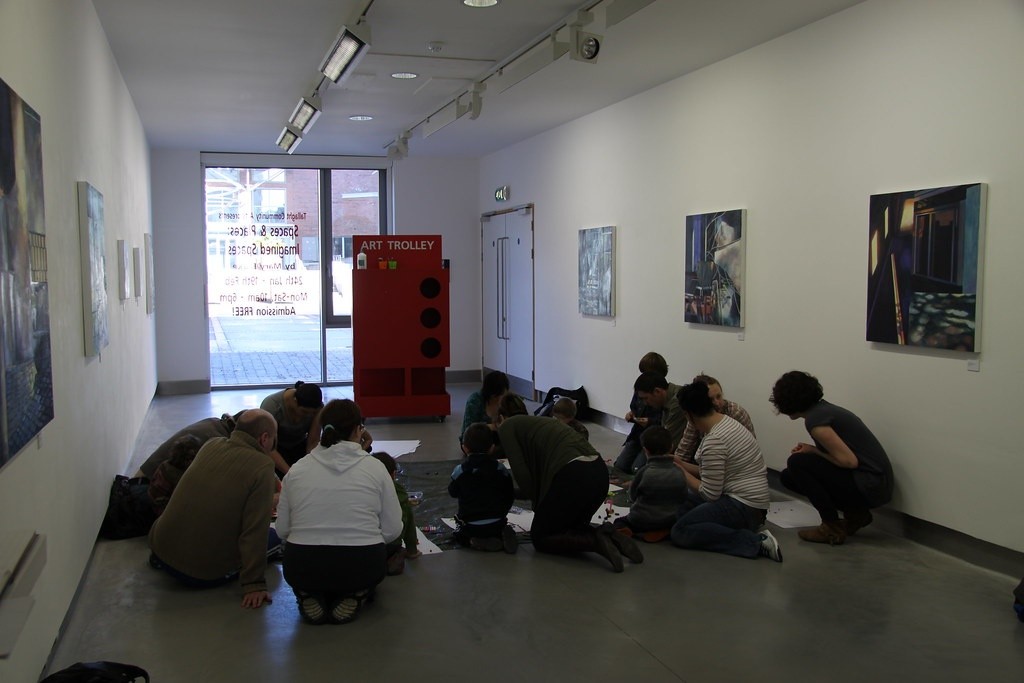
[757,529,783,562]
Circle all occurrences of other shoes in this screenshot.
[299,588,329,624]
[470,537,503,552]
[594,527,623,573]
[330,567,376,622]
[598,522,643,563]
[501,525,518,553]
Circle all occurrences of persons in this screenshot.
[459,371,510,460]
[671,382,783,563]
[134,409,248,478]
[492,393,644,573]
[674,375,755,464]
[614,425,688,543]
[275,398,404,625]
[360,427,372,453]
[148,406,282,609]
[553,397,589,440]
[770,371,893,545]
[260,381,324,480]
[447,423,518,554]
[372,452,421,575]
[147,434,204,514]
[614,352,683,475]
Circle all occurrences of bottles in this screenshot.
[357,249,367,269]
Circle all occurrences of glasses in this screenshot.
[359,424,365,433]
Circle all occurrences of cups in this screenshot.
[377,258,387,269]
[388,256,397,269]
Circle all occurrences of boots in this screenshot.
[845,508,873,533]
[797,518,846,545]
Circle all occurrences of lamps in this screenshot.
[467,83,487,120]
[318,16,372,87]
[567,11,604,64]
[394,131,413,157]
[276,126,303,155]
[497,31,571,96]
[423,98,466,139]
[288,90,323,135]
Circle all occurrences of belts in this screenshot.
[126,477,152,487]
